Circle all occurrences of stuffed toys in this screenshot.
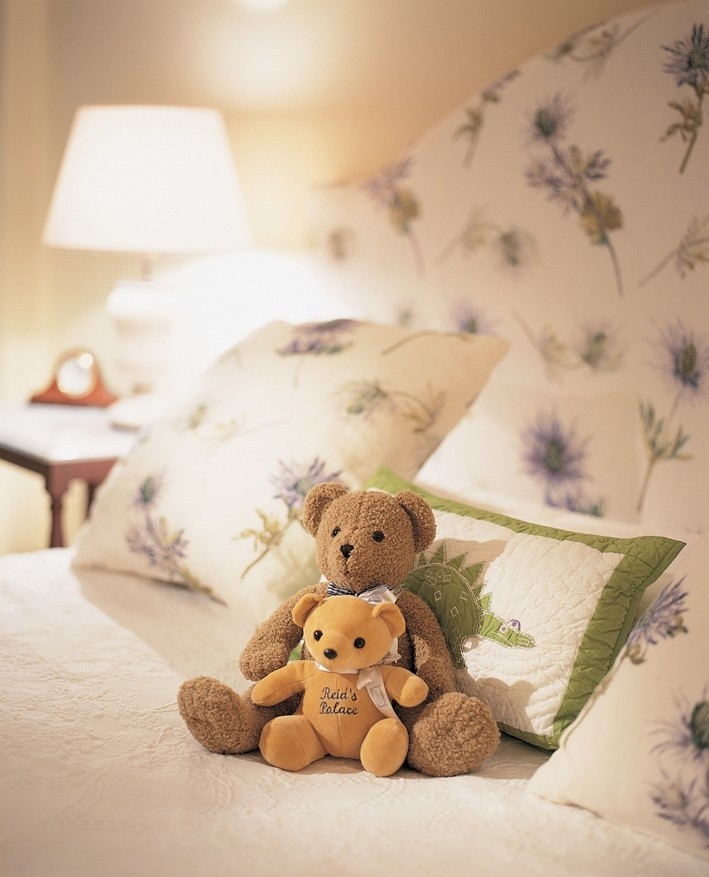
[176,480,499,778]
[252,584,431,776]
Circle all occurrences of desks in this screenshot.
[0,404,145,550]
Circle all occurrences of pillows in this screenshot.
[71,318,709,862]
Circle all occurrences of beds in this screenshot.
[0,0,709,877]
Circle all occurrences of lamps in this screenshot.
[43,104,253,428]
